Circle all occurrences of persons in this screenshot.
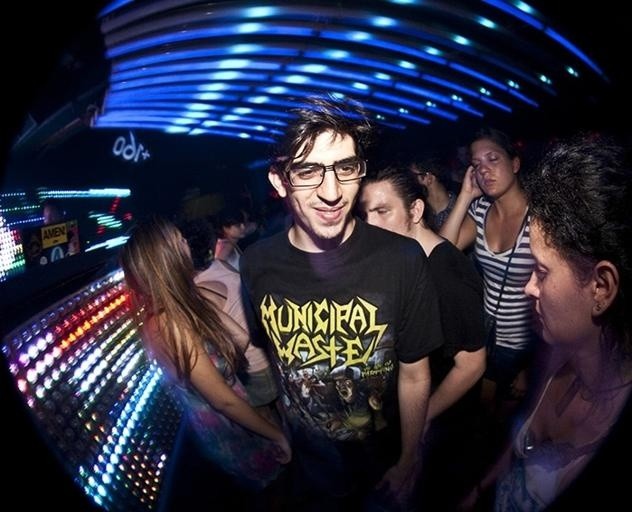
[121,97,632,512]
[41,198,67,225]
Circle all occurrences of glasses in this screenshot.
[282,155,367,187]
